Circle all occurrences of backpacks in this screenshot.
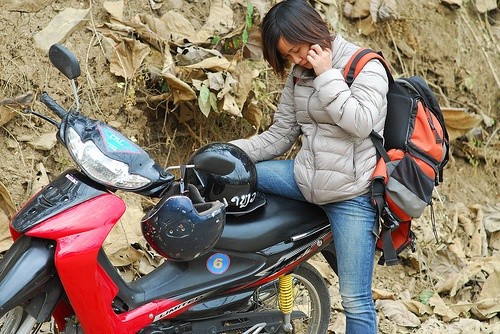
[342,48,449,267]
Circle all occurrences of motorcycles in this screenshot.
[0,44,336,334]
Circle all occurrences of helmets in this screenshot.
[184,143,267,215]
[141,183,226,263]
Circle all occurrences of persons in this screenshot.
[228,0,389,333]
